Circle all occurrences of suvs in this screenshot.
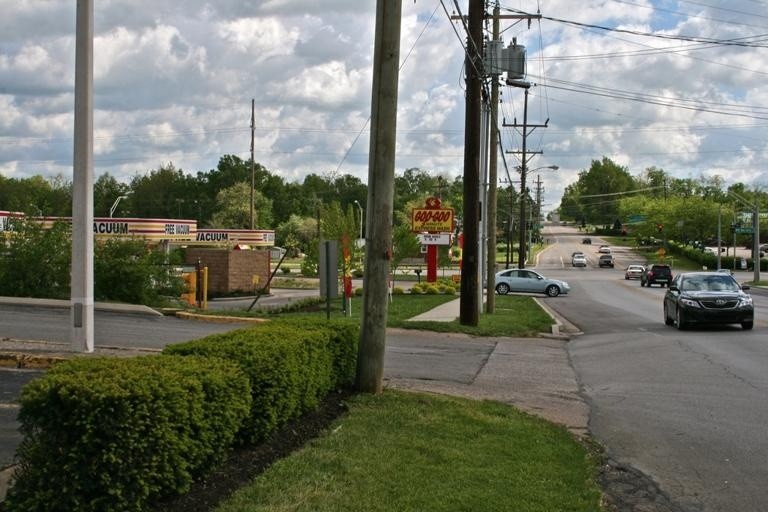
[639,264,672,287]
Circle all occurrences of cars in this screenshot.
[599,254,615,268]
[624,265,646,280]
[662,270,756,330]
[493,269,570,297]
[598,245,610,253]
[571,252,587,266]
[680,237,768,257]
[582,238,591,244]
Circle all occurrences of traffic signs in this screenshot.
[734,228,754,234]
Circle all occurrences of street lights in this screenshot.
[109,195,128,219]
[353,200,363,267]
[197,257,203,308]
[175,199,185,217]
[704,189,761,284]
[194,200,202,229]
[520,165,559,270]
